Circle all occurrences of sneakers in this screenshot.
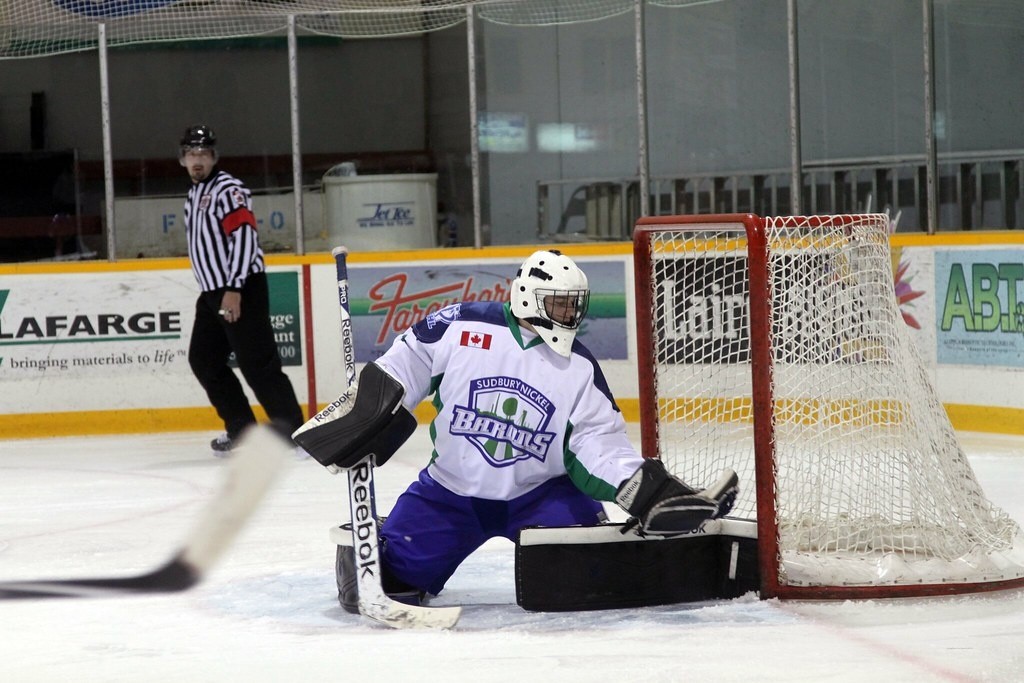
[211,415,257,454]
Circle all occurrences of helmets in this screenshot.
[509,249,591,358]
[178,125,217,150]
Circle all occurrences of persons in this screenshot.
[291,250,760,611]
[178,124,304,451]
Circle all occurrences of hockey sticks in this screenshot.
[0,422,296,603]
[330,244,464,630]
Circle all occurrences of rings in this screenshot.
[225,310,232,314]
